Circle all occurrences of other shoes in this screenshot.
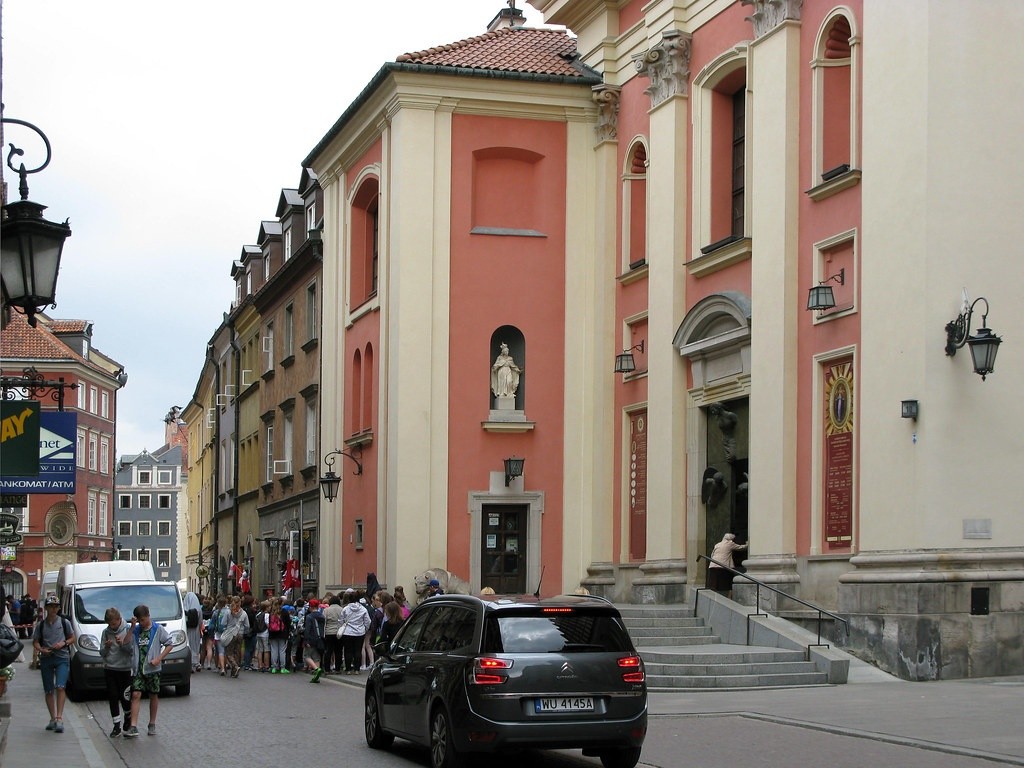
[46,720,56,730]
[110,727,121,738]
[56,721,64,731]
[123,717,131,732]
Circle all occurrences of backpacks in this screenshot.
[269,613,284,631]
[184,608,199,628]
[256,611,268,633]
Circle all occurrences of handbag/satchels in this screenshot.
[337,623,347,639]
[0,624,25,669]
[220,626,235,647]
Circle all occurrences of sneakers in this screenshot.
[147,723,157,734]
[123,726,139,737]
[191,663,374,681]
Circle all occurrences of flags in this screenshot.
[228,561,238,576]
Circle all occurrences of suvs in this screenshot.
[363,592,649,768]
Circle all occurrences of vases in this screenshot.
[0,676,7,697]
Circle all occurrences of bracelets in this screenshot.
[64,640,68,646]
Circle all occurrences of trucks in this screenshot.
[55,559,200,703]
[37,570,60,619]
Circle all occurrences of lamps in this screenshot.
[79,545,99,562]
[111,541,123,550]
[613,339,644,374]
[944,297,1003,383]
[502,454,525,488]
[805,267,845,311]
[900,399,918,423]
[319,440,363,503]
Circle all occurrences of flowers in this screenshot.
[0,664,17,681]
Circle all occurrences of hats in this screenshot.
[45,596,60,606]
[309,598,318,607]
[426,580,439,586]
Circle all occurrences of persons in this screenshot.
[424,580,444,599]
[221,596,250,678]
[194,586,413,675]
[303,599,325,683]
[268,598,294,674]
[709,402,739,464]
[491,342,525,397]
[322,598,343,675]
[183,592,206,672]
[480,586,496,596]
[100,607,132,739]
[377,601,405,646]
[706,471,727,508]
[566,586,591,644]
[337,592,372,675]
[0,579,44,638]
[32,596,76,731]
[122,605,174,736]
[709,533,748,599]
